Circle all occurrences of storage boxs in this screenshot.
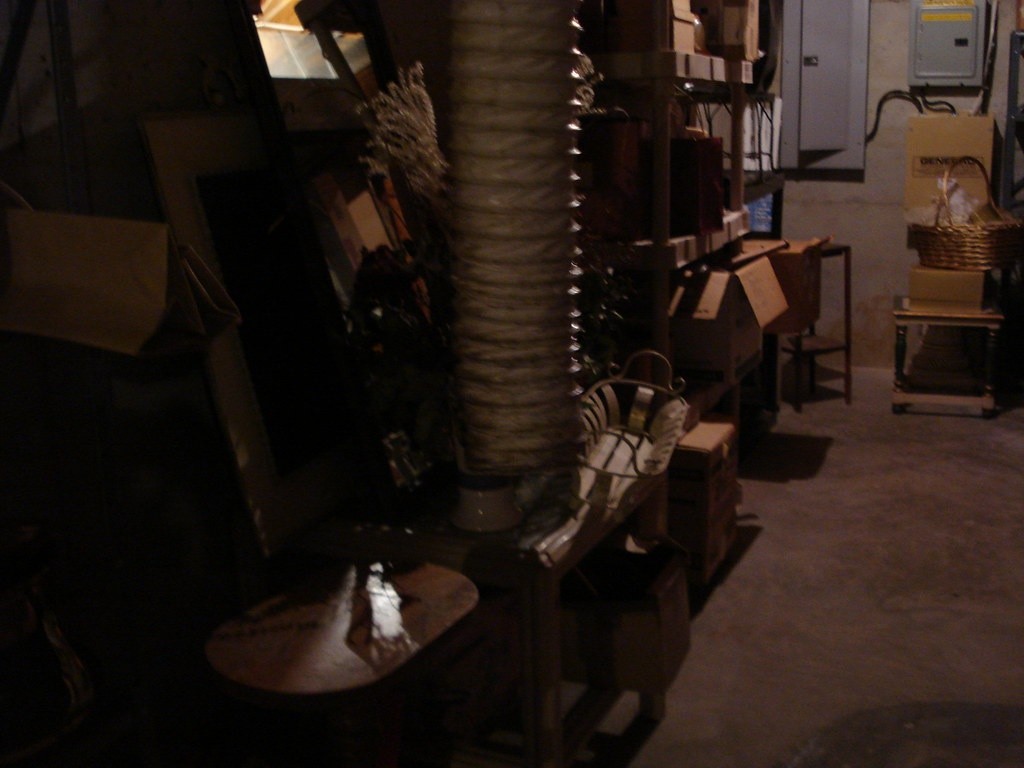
[664,411,740,584]
[726,237,831,335]
[666,255,791,372]
[567,0,760,239]
[908,261,985,315]
[443,572,699,752]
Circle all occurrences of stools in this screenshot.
[890,292,1005,420]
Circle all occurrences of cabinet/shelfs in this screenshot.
[777,243,853,413]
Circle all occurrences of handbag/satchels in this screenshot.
[640,96,724,236]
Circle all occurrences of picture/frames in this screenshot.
[133,102,374,557]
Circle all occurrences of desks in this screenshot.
[206,550,479,767]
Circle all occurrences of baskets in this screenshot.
[908,157,1024,271]
